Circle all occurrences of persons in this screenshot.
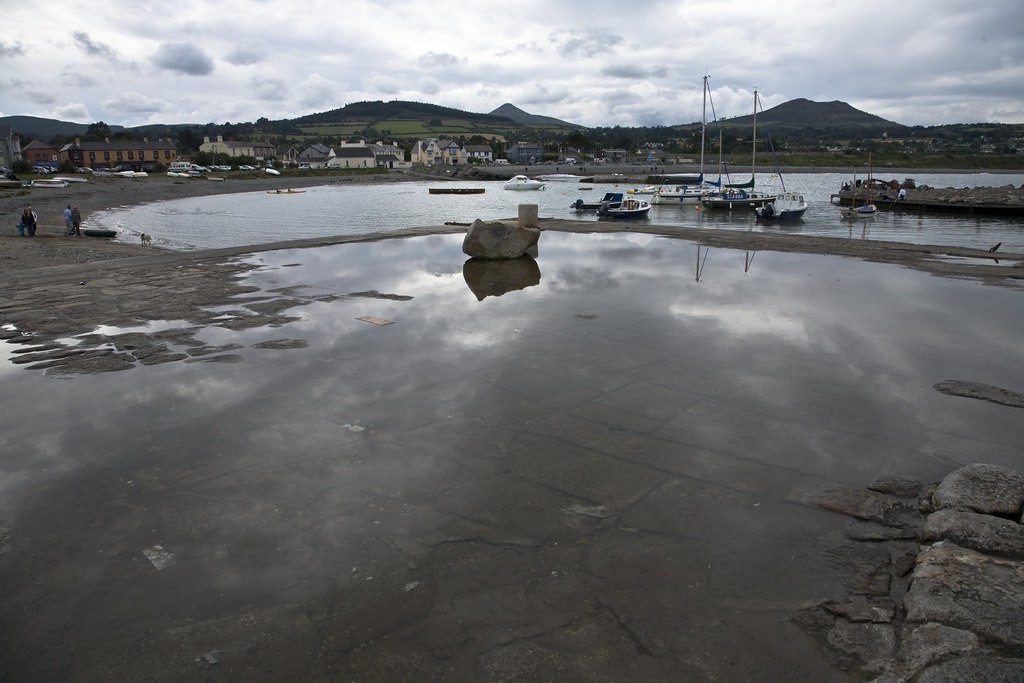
[63,205,81,236]
[661,167,665,174]
[18,206,38,238]
[579,166,586,173]
[276,188,280,193]
[632,167,634,174]
[650,165,658,174]
[524,167,528,172]
[642,169,645,174]
[288,187,291,192]
[556,167,559,172]
[897,188,906,202]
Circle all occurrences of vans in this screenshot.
[495,159,508,164]
[168,162,191,172]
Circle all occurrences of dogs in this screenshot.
[140,232,152,247]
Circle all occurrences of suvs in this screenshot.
[328,163,341,168]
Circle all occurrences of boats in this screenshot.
[113,171,149,177]
[841,152,878,218]
[267,190,306,193]
[261,168,280,175]
[503,175,546,190]
[22,177,88,187]
[84,229,117,237]
[570,193,651,218]
[429,188,485,194]
[536,174,612,183]
[168,170,224,181]
[626,66,808,220]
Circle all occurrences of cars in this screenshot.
[191,164,273,173]
[33,166,58,174]
[299,165,313,169]
[100,168,119,173]
[78,167,93,173]
